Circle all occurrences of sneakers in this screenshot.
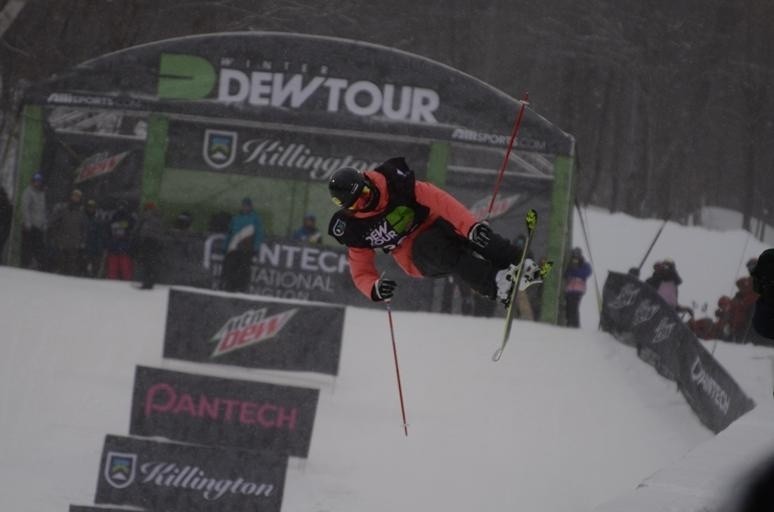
[495,264,516,306]
[518,258,537,292]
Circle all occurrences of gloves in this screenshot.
[372,278,397,302]
[469,222,490,248]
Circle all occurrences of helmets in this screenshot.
[328,167,378,212]
[32,173,43,182]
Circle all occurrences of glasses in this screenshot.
[347,183,373,211]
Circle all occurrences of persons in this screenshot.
[18,173,50,272]
[630,247,773,347]
[292,212,323,247]
[133,200,162,289]
[442,233,592,329]
[157,213,198,287]
[327,154,551,314]
[41,187,84,277]
[71,198,102,279]
[220,196,264,293]
[105,198,138,279]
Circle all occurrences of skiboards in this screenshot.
[492,209,555,360]
